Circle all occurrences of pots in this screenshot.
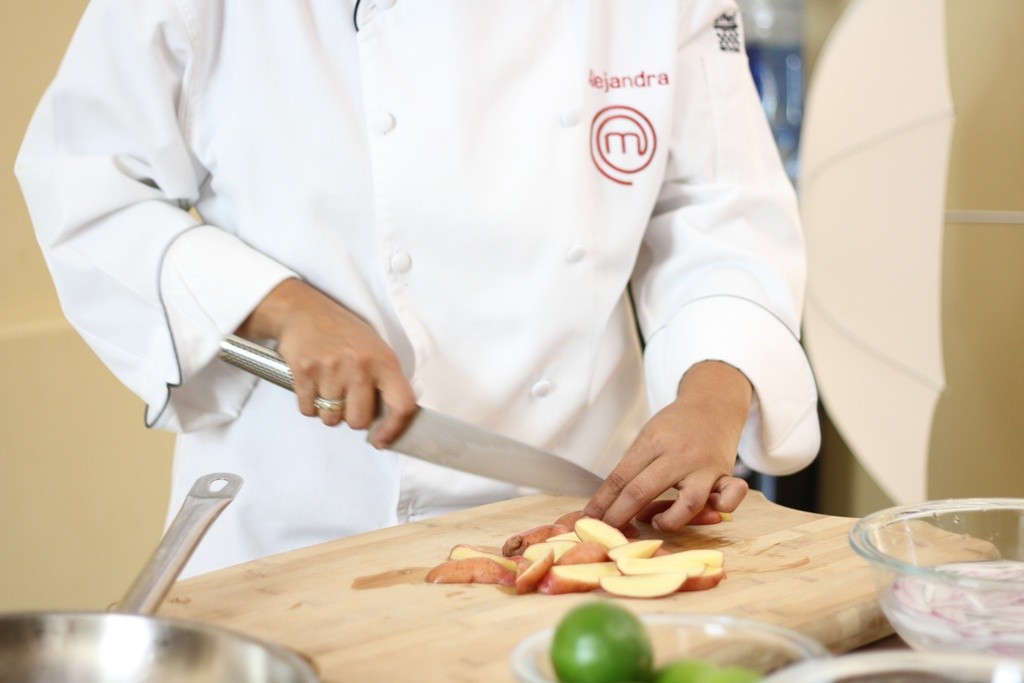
[0,471,324,683]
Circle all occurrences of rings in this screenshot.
[313,396,344,412]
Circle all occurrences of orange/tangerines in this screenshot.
[553,603,764,683]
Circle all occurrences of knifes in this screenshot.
[212,332,603,496]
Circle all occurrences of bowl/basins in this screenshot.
[511,479,1022,682]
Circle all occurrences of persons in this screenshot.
[8,1,823,577]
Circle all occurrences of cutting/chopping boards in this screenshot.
[113,478,1003,680]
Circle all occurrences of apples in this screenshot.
[427,515,726,599]
